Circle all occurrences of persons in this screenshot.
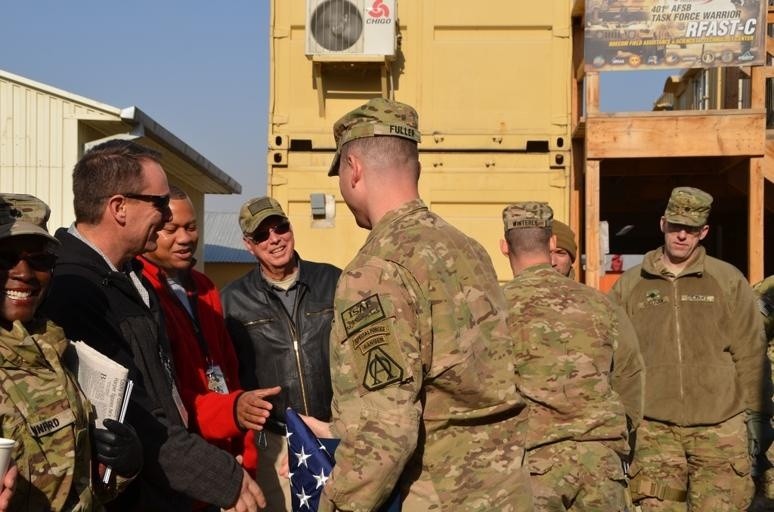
[32,138,269,512]
[136,182,283,481]
[495,201,647,512]
[315,96,533,512]
[216,195,345,512]
[606,185,765,511]
[0,458,19,511]
[0,191,145,512]
[548,219,579,281]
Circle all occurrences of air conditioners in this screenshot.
[305,0,397,61]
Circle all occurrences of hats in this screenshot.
[550,217,579,264]
[328,99,421,178]
[502,201,554,231]
[238,196,289,234]
[0,192,57,245]
[664,188,713,228]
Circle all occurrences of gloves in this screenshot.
[90,417,143,480]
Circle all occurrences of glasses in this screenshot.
[120,190,174,210]
[2,246,61,276]
[246,221,293,244]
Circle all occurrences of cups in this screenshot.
[0,436,19,497]
